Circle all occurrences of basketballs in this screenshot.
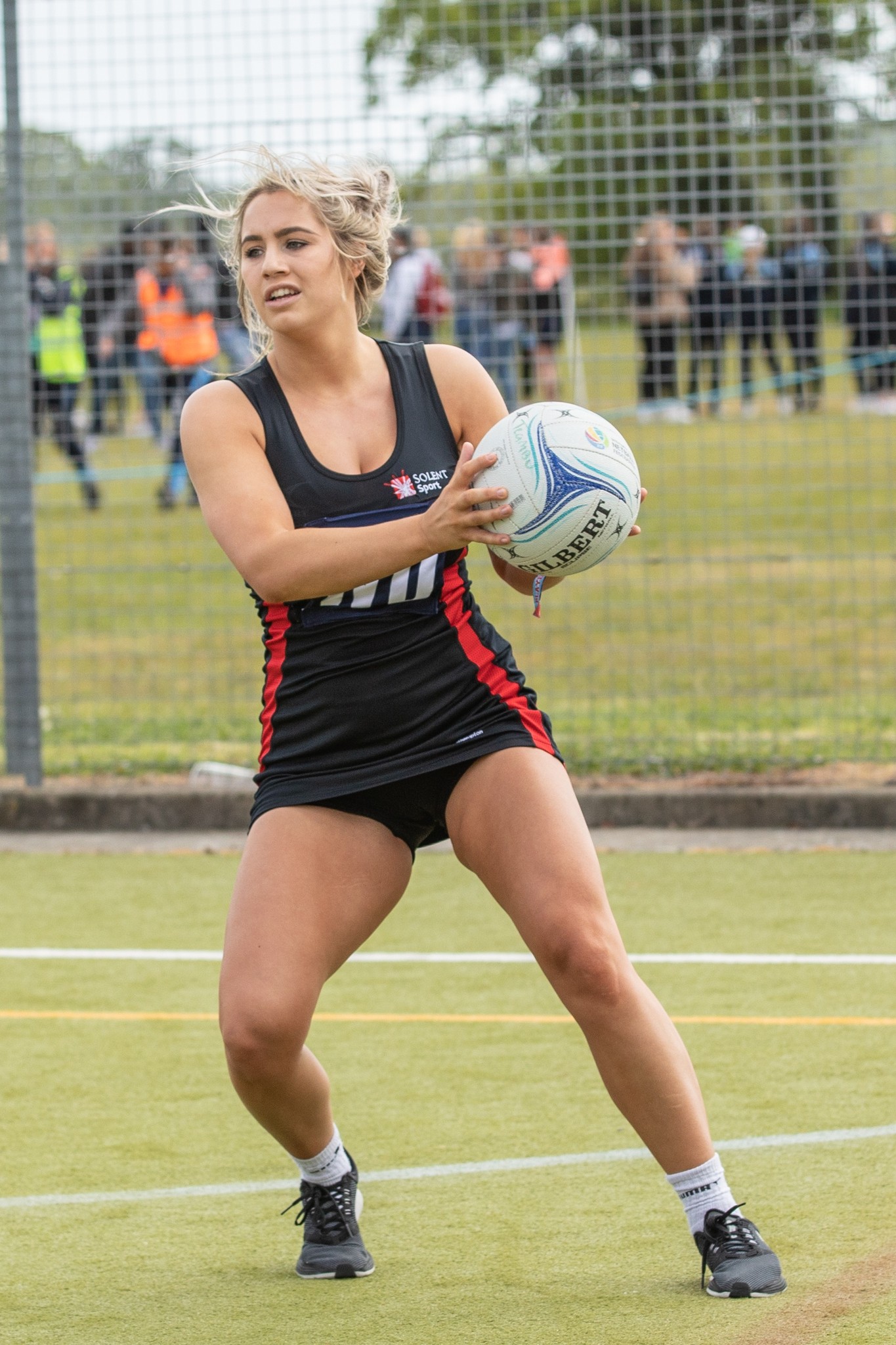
[467,401,639,577]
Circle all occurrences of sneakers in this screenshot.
[686,1202,788,1298]
[280,1147,377,1278]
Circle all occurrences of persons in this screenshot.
[22,210,896,510]
[175,154,789,1297]
